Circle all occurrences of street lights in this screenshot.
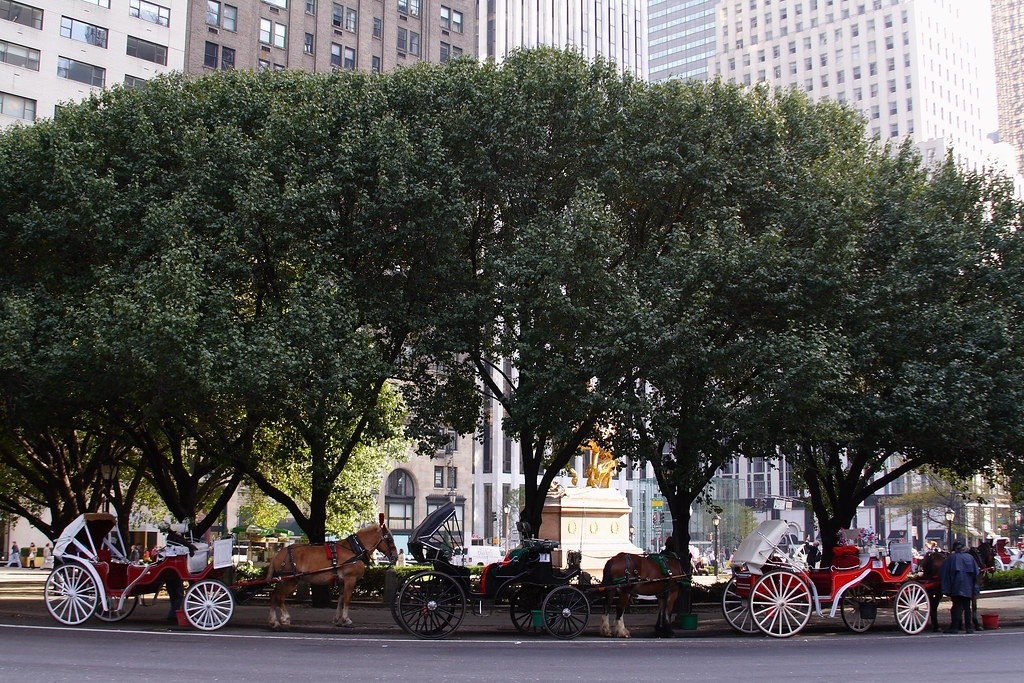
[712,515,721,576]
[503,502,511,554]
[629,525,635,544]
[945,507,955,554]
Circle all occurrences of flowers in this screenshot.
[836,526,877,548]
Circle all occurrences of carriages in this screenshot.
[721,520,997,640]
[390,502,692,640]
[43,513,399,631]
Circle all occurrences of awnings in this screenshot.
[925,530,945,540]
[888,531,907,540]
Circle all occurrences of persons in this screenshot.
[43,544,51,563]
[397,549,405,566]
[4,541,22,569]
[28,542,37,569]
[693,547,737,575]
[130,545,139,562]
[805,540,821,568]
[938,542,979,634]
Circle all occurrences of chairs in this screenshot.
[515,522,560,548]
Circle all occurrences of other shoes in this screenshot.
[943,629,958,633]
[966,629,973,633]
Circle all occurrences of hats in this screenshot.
[46,543,49,546]
[951,541,965,551]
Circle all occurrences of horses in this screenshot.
[266,521,398,632]
[922,539,996,632]
[600,536,694,637]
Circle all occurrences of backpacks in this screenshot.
[815,549,821,562]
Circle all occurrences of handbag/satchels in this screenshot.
[29,551,34,560]
[396,560,400,566]
[726,560,731,569]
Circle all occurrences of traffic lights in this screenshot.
[660,511,664,524]
[653,516,657,524]
[491,511,497,522]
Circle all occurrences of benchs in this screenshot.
[760,557,811,590]
[426,549,471,590]
[997,548,1011,563]
[491,549,529,577]
[810,547,860,595]
[81,550,129,577]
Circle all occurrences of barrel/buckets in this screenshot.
[858,601,877,619]
[531,610,550,627]
[176,609,193,626]
[980,613,999,629]
[679,614,698,630]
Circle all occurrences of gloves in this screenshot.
[189,544,198,557]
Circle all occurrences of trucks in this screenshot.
[452,546,510,567]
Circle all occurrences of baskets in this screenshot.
[859,602,877,619]
[532,610,550,626]
[981,614,998,628]
[680,614,697,629]
[176,610,195,625]
